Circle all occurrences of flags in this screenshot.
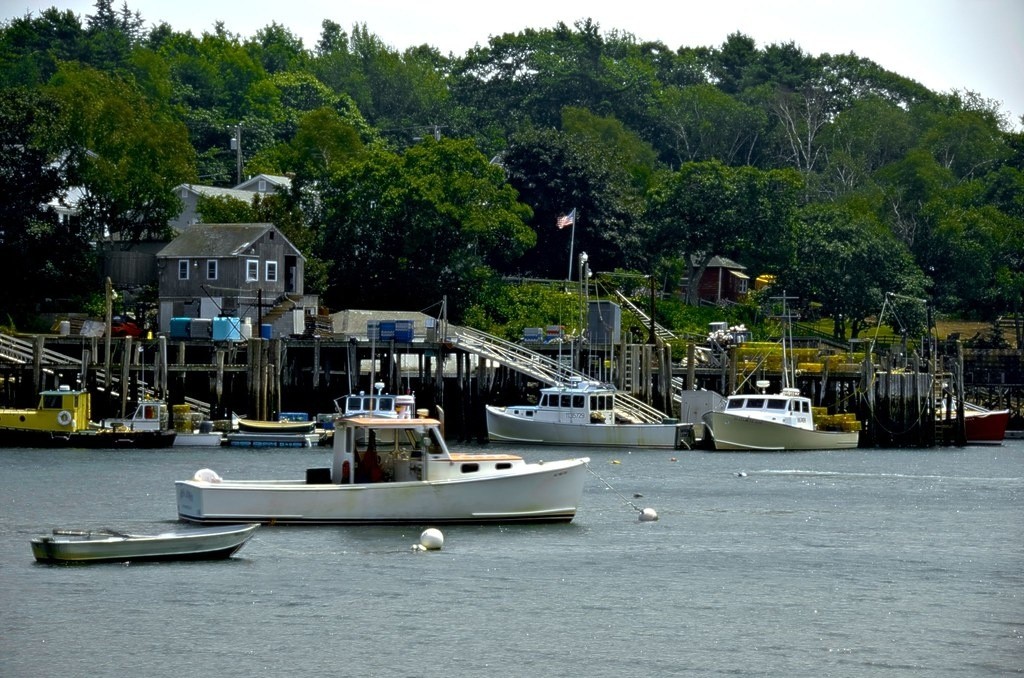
[557,209,574,230]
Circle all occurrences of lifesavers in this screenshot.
[57,411,72,425]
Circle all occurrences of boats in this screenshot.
[0,385,178,450]
[175,312,591,526]
[174,379,415,446]
[485,301,696,451]
[935,393,1010,445]
[28,518,261,566]
[701,289,861,452]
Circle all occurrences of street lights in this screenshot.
[578,250,589,345]
